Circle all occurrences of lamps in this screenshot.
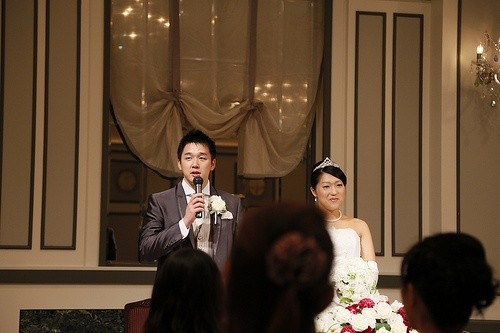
[470,30,500,112]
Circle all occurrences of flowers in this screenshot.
[207,195,227,224]
[319,257,412,333]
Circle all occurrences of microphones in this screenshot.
[193,175,203,218]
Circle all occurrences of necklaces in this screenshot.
[324,210,342,221]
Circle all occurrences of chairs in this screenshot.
[124,299,152,333]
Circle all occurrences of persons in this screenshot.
[311,156,376,262]
[401,232,500,333]
[138,129,247,286]
[225,202,333,333]
[143,248,223,333]
